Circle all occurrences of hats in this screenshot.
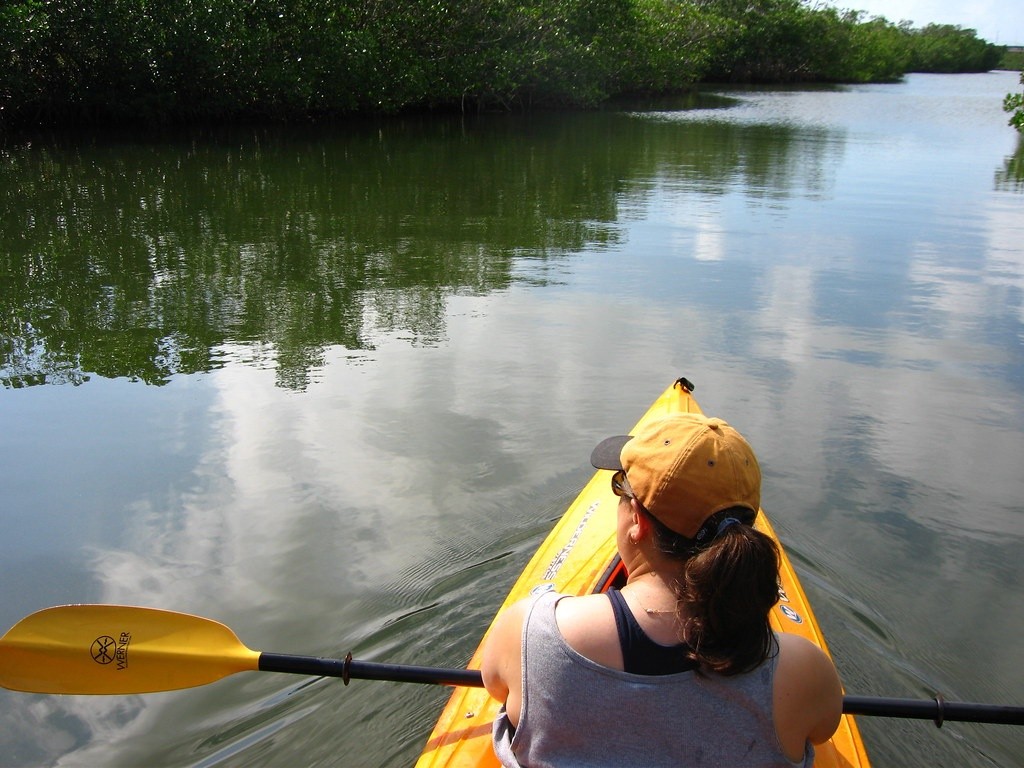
[590,413,761,543]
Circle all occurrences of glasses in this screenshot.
[612,471,646,512]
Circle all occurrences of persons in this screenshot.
[481,412,843,768]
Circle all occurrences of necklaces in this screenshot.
[627,584,677,614]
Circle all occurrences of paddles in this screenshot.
[0,602,1024,729]
